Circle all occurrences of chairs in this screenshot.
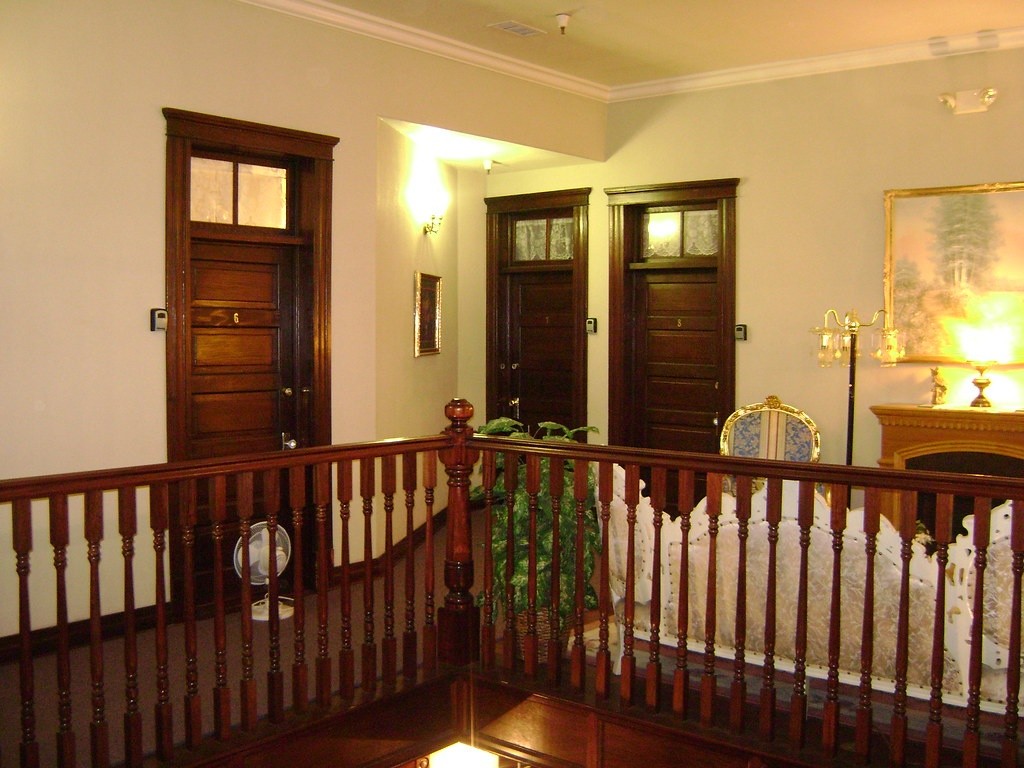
[718,395,821,499]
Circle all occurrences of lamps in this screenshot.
[965,359,998,408]
[423,200,446,236]
[808,308,904,510]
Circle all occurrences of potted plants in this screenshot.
[477,417,611,665]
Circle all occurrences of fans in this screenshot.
[233,522,295,621]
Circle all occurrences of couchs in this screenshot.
[590,444,1023,757]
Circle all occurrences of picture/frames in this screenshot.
[884,180,1023,371]
[413,270,443,357]
